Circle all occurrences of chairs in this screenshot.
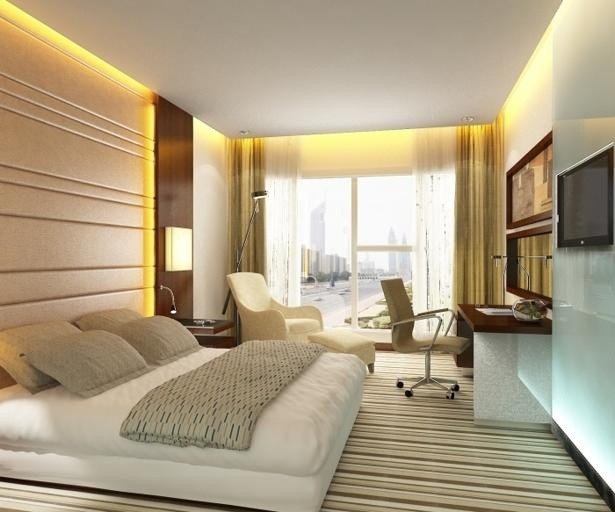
[379,277,472,400]
[225,272,323,343]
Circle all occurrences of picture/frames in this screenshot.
[505,130,553,229]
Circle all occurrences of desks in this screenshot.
[453,304,552,433]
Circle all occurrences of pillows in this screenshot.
[0,319,84,395]
[17,330,156,400]
[71,309,144,332]
[111,315,205,366]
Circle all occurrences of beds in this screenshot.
[0,326,369,512]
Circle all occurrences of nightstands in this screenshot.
[173,318,236,347]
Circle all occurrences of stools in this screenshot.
[309,328,377,374]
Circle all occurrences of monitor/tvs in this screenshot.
[555,140,615,249]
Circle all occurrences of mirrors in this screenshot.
[506,225,553,310]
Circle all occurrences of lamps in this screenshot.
[222,190,268,347]
[165,226,193,272]
[160,285,177,314]
[518,255,552,291]
[491,255,507,306]
